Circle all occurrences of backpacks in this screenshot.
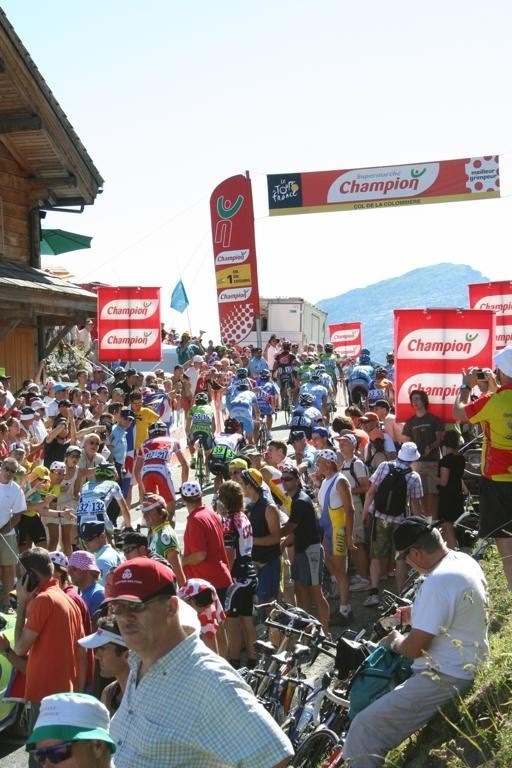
[374,460,413,517]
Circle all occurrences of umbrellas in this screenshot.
[39,229,92,256]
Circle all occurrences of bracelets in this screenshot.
[58,512,60,518]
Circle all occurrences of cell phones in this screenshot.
[22,569,39,592]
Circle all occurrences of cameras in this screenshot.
[475,370,490,382]
[66,482,72,486]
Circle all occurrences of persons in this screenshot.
[419,429,466,552]
[68,551,105,618]
[282,467,332,650]
[183,356,204,418]
[360,442,424,607]
[184,335,291,512]
[161,321,214,365]
[402,390,445,529]
[335,434,369,590]
[335,348,395,436]
[79,464,134,550]
[135,494,186,586]
[341,518,490,768]
[106,410,135,527]
[0,457,27,614]
[0,365,184,481]
[24,693,117,768]
[229,459,295,607]
[134,421,189,529]
[77,617,131,719]
[452,346,512,588]
[183,361,208,372]
[124,534,174,570]
[64,317,98,365]
[21,465,57,551]
[291,342,335,487]
[0,551,95,692]
[14,548,87,736]
[239,467,281,641]
[45,445,82,556]
[374,399,411,444]
[99,557,295,768]
[39,461,78,520]
[174,482,234,657]
[81,521,125,587]
[309,449,354,626]
[359,412,397,460]
[216,481,259,670]
[369,430,388,474]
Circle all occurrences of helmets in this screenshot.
[261,369,271,379]
[298,393,314,403]
[360,347,370,356]
[359,354,370,364]
[309,373,322,384]
[304,357,315,365]
[148,422,169,438]
[323,342,333,352]
[314,364,325,374]
[235,378,250,390]
[375,366,389,379]
[282,340,293,351]
[223,418,239,433]
[236,368,248,376]
[194,393,208,404]
[92,460,118,481]
[387,352,394,364]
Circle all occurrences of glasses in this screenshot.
[122,418,132,422]
[27,743,77,764]
[3,467,15,475]
[37,478,47,484]
[52,470,66,477]
[289,438,303,444]
[66,453,80,459]
[131,402,142,406]
[279,475,296,482]
[87,441,99,447]
[123,546,141,555]
[108,598,164,617]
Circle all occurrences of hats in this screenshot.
[31,399,49,410]
[0,389,7,397]
[192,354,205,364]
[390,514,441,565]
[241,468,263,488]
[283,466,302,490]
[25,383,40,392]
[368,400,390,410]
[98,556,177,601]
[240,445,262,459]
[49,551,69,569]
[369,429,385,443]
[0,374,12,381]
[25,691,119,756]
[219,479,244,508]
[335,433,358,447]
[54,383,69,395]
[113,366,126,376]
[359,412,379,423]
[57,399,76,409]
[74,521,106,543]
[396,441,421,462]
[27,465,52,484]
[312,426,331,439]
[66,552,101,575]
[135,493,168,513]
[286,431,305,445]
[76,624,127,657]
[126,368,139,377]
[50,461,66,471]
[174,481,202,497]
[318,448,338,467]
[19,411,40,420]
[492,344,512,379]
[229,459,247,471]
[120,409,135,421]
[66,445,82,455]
[115,532,150,546]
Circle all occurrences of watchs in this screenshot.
[460,384,472,391]
[390,637,400,653]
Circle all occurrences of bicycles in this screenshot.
[243,589,410,768]
[284,396,291,424]
[259,412,277,452]
[192,433,207,491]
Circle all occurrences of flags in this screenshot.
[170,281,190,314]
